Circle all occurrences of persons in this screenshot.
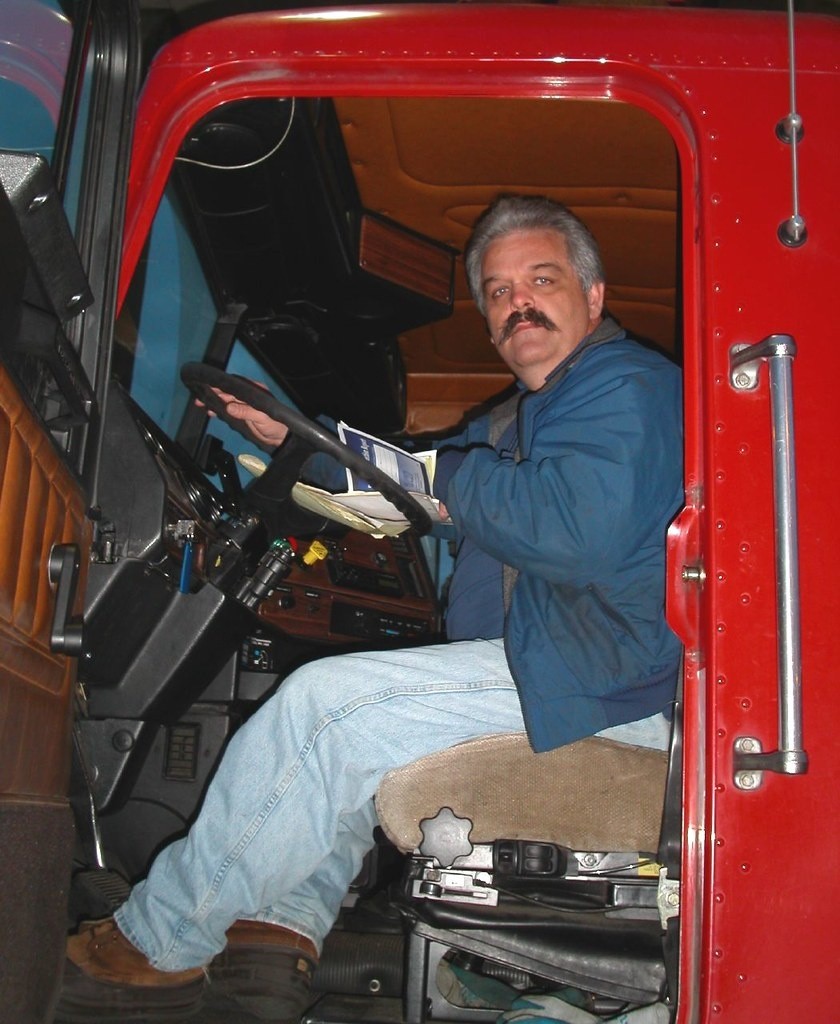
[62,191,684,1016]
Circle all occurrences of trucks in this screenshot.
[0,0,839,1024]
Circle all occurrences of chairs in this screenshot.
[374,728,671,1023]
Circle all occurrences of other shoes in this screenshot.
[61,916,205,1023]
[205,920,320,1023]
